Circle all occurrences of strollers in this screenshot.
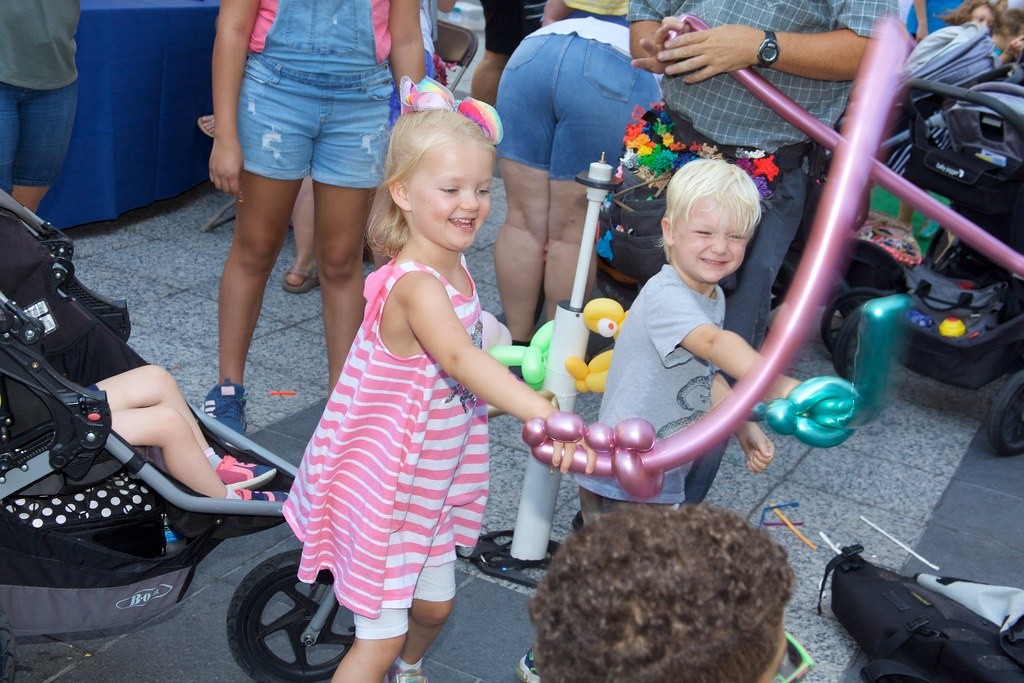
[819,64,1024,456]
[594,103,831,307]
[1,187,356,683]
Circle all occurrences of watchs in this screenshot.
[757,26,780,68]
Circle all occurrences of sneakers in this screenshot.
[516,649,542,683]
[200,378,248,435]
[215,455,277,491]
[234,487,289,502]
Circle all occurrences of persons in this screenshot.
[529,508,796,683]
[203,0,1024,439]
[280,76,595,683]
[21,364,291,504]
[0,0,81,224]
[515,155,810,683]
[573,0,902,540]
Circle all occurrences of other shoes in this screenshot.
[385,664,428,683]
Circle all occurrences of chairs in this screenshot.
[198,19,478,232]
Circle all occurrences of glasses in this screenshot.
[776,631,814,683]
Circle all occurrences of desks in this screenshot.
[35,0,221,229]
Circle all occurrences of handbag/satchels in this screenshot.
[599,109,737,295]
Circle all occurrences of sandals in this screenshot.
[282,265,320,294]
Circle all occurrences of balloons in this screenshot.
[764,294,908,447]
[486,315,557,391]
[519,15,907,503]
[683,17,1024,277]
[564,297,629,395]
